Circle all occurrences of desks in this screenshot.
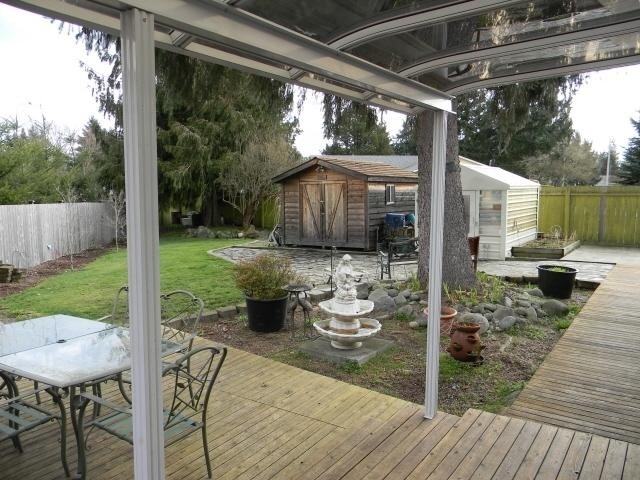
[0,314,188,389]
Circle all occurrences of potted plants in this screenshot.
[233,253,313,333]
[536,264,579,300]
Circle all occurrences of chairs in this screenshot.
[97,286,129,327]
[160,290,205,408]
[70,345,227,479]
[0,386,70,479]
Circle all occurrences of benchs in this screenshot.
[375,227,420,279]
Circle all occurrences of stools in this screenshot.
[287,284,311,341]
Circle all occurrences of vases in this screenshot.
[424,306,486,367]
